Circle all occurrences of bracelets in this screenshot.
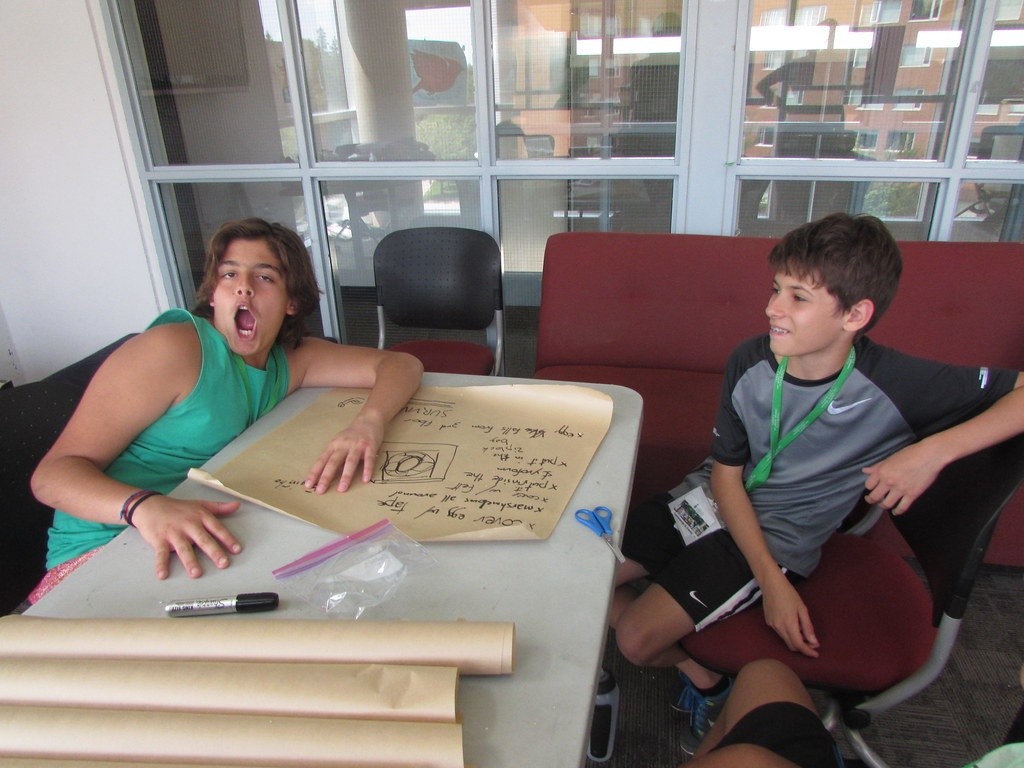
[120,490,163,528]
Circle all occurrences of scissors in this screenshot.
[575,508,628,564]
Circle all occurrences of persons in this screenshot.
[681,660,1024,768]
[30,218,424,579]
[754,20,854,233]
[618,11,685,218]
[608,213,1024,756]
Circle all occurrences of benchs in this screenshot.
[536,232,1024,567]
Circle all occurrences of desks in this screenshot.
[0,371,646,768]
[275,147,434,269]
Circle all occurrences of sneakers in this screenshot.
[668,684,702,726]
[679,677,735,754]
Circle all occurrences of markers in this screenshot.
[164,591,278,621]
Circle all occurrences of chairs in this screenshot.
[0,332,142,617]
[675,432,1024,768]
[373,227,503,376]
